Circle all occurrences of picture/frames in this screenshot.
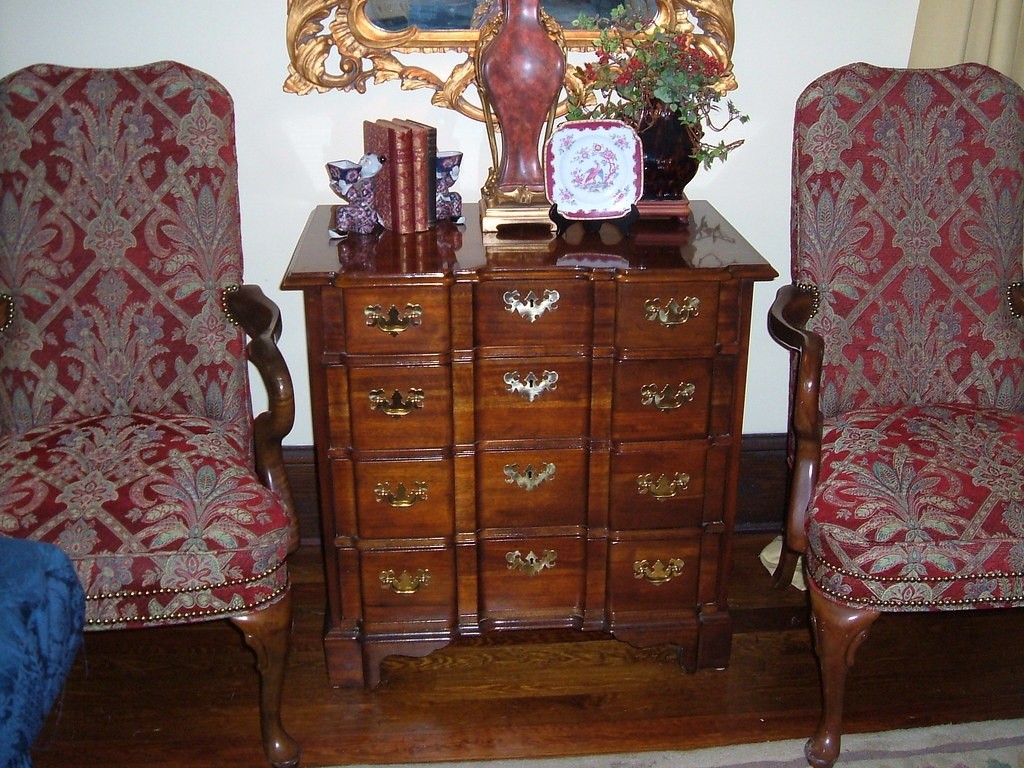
[283,0,739,134]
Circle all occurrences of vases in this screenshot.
[619,97,705,200]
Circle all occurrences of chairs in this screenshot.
[0,60,302,768]
[768,63,1024,768]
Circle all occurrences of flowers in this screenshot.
[565,5,750,170]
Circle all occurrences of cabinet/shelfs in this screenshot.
[279,201,779,689]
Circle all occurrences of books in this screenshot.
[361,119,440,236]
